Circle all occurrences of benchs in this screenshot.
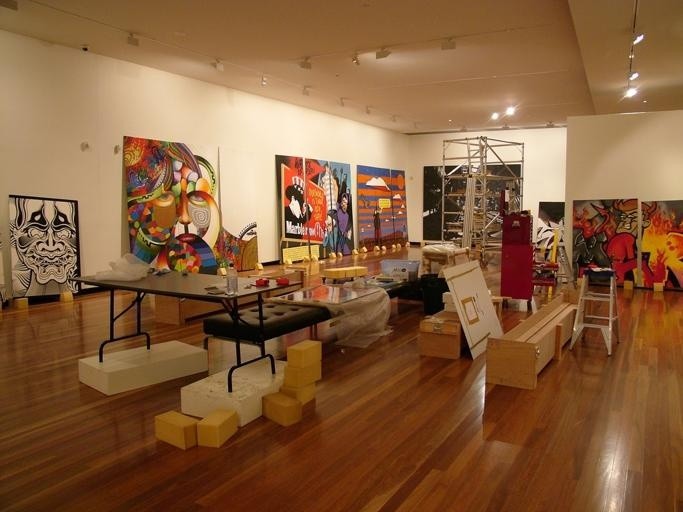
[202,279,411,377]
[419,240,469,275]
[154,265,305,325]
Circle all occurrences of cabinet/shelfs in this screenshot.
[485,291,591,391]
[417,296,502,360]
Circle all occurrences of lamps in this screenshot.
[296,35,459,70]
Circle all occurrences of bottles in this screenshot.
[391,267,408,282]
[502,297,509,311]
[226,263,238,297]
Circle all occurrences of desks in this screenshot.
[66,270,302,394]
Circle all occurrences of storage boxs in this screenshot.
[379,259,420,282]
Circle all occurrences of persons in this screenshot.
[335,192,353,255]
[321,209,339,251]
[284,182,313,248]
